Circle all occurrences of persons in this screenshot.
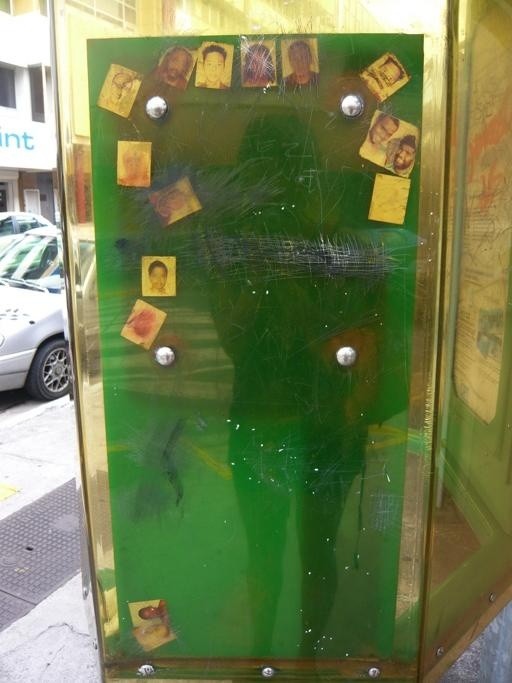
[136,601,165,625]
[102,72,131,112]
[158,48,190,87]
[364,114,401,164]
[287,42,315,86]
[369,60,406,92]
[244,46,274,88]
[147,261,168,297]
[385,134,417,176]
[199,44,230,89]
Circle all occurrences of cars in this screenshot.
[0,207,74,402]
